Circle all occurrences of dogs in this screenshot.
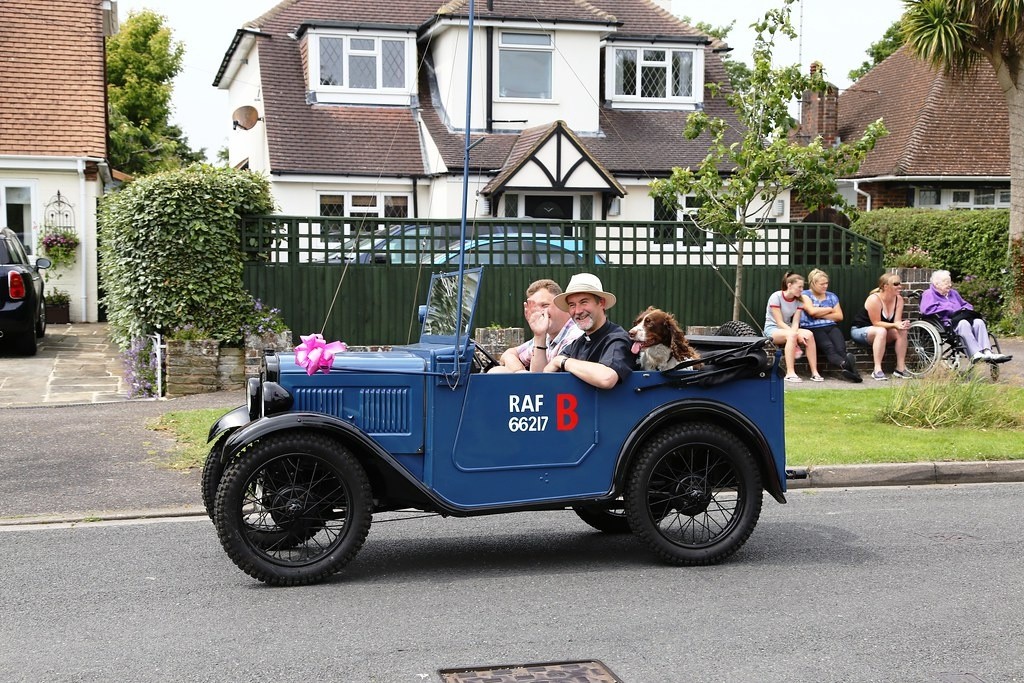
[628,306,703,372]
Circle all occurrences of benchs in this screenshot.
[628,339,753,371]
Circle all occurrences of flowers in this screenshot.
[890,247,933,270]
[33,220,80,280]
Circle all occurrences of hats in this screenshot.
[552,273,616,312]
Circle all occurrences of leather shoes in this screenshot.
[842,370,863,383]
[839,353,857,373]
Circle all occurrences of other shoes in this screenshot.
[893,366,915,379]
[986,352,1003,360]
[971,352,990,361]
[871,370,888,381]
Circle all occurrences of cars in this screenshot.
[419,233,608,264]
[201,264,808,586]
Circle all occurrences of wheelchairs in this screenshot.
[904,289,1012,382]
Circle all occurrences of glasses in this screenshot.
[889,282,901,286]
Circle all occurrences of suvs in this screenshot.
[310,216,562,264]
[0,228,52,357]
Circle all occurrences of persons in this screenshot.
[919,270,1005,360]
[798,268,863,383]
[523,272,633,390]
[763,270,824,383]
[489,279,585,374]
[851,273,913,381]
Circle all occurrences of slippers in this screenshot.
[784,375,803,383]
[810,375,825,382]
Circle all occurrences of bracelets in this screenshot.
[561,358,567,372]
[536,346,547,350]
